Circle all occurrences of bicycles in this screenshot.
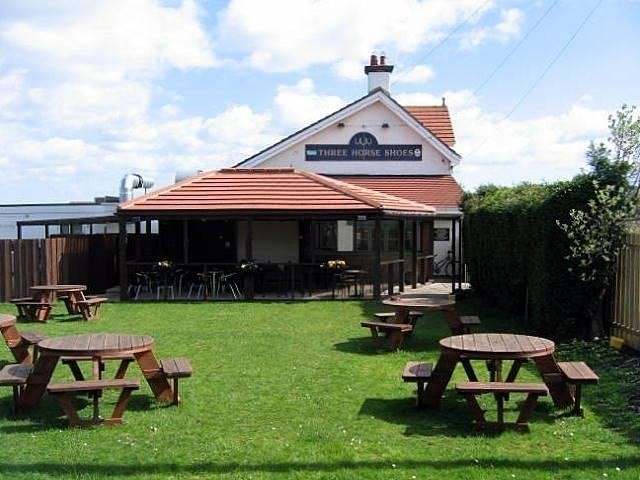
[430,250,461,280]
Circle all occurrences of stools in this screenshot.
[557,361,599,416]
[19,330,48,363]
[459,315,481,334]
[0,364,35,417]
[160,357,192,406]
[402,361,433,409]
[47,379,139,429]
[61,354,136,381]
[360,320,413,348]
[373,310,424,334]
[7,284,108,322]
[456,381,549,432]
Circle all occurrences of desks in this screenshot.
[19,334,174,408]
[381,297,465,344]
[422,333,575,408]
[0,313,33,364]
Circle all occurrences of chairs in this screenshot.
[127,269,241,300]
[249,258,367,298]
